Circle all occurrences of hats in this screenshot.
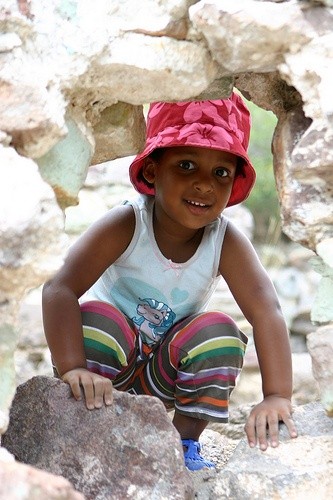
[129,92,256,208]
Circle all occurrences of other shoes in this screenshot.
[182,439,215,471]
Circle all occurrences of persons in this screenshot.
[43,92,298,470]
